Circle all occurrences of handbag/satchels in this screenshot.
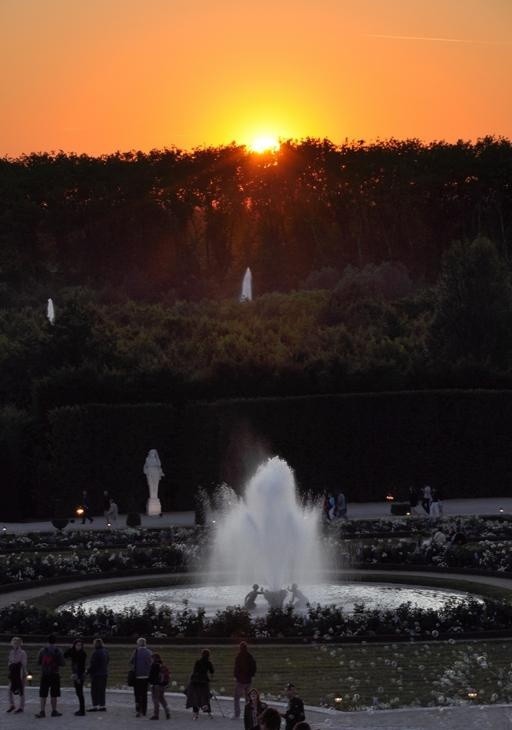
[128,671,138,686]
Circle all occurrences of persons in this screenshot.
[408,483,440,518]
[81,489,119,524]
[232,641,257,718]
[36,635,66,718]
[182,649,215,720]
[287,583,309,607]
[243,683,311,730]
[148,654,171,721]
[324,490,347,522]
[143,449,165,498]
[244,584,264,608]
[85,638,109,712]
[64,639,87,716]
[129,638,154,718]
[6,637,28,715]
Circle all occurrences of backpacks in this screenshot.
[159,665,170,686]
